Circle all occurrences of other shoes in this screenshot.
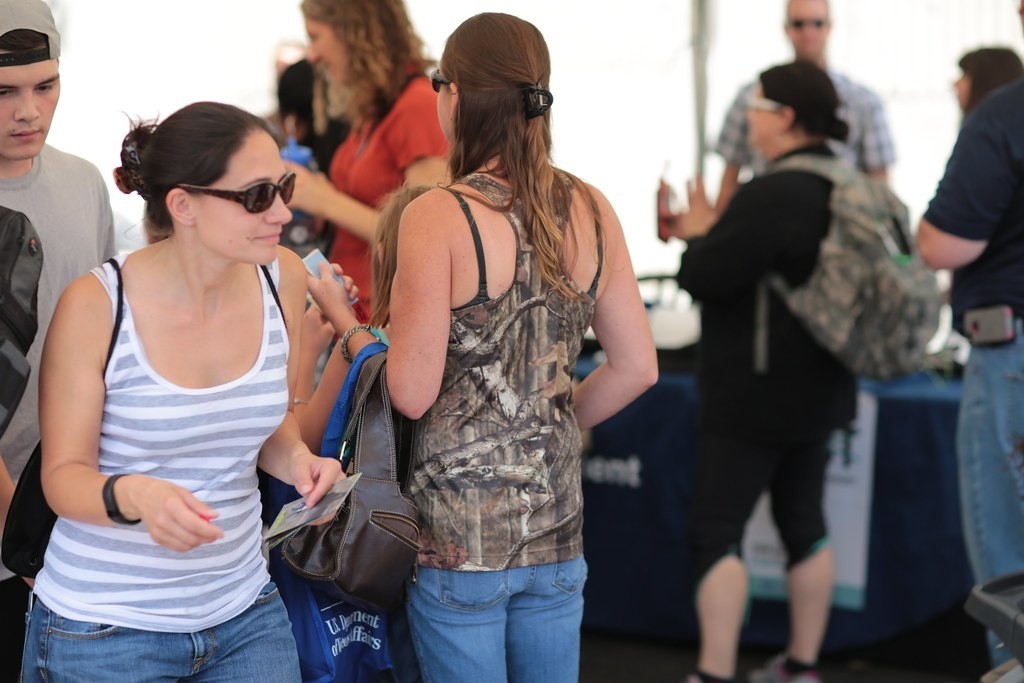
[749,652,818,683]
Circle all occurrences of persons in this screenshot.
[919,77,1024,666]
[714,0,896,211]
[308,13,658,683]
[272,0,449,683]
[18,100,346,683]
[660,64,856,683]
[954,48,1024,114]
[0,0,117,682]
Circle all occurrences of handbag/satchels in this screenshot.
[265,342,395,683]
[278,350,421,612]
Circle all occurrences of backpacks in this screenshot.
[749,156,957,382]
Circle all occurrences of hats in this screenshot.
[0,0,62,66]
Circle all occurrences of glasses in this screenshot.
[737,92,781,114]
[790,18,824,29]
[431,69,453,93]
[177,171,297,213]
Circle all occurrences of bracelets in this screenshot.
[340,326,372,363]
[103,474,142,525]
[294,398,307,404]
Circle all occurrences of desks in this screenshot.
[571,361,988,663]
